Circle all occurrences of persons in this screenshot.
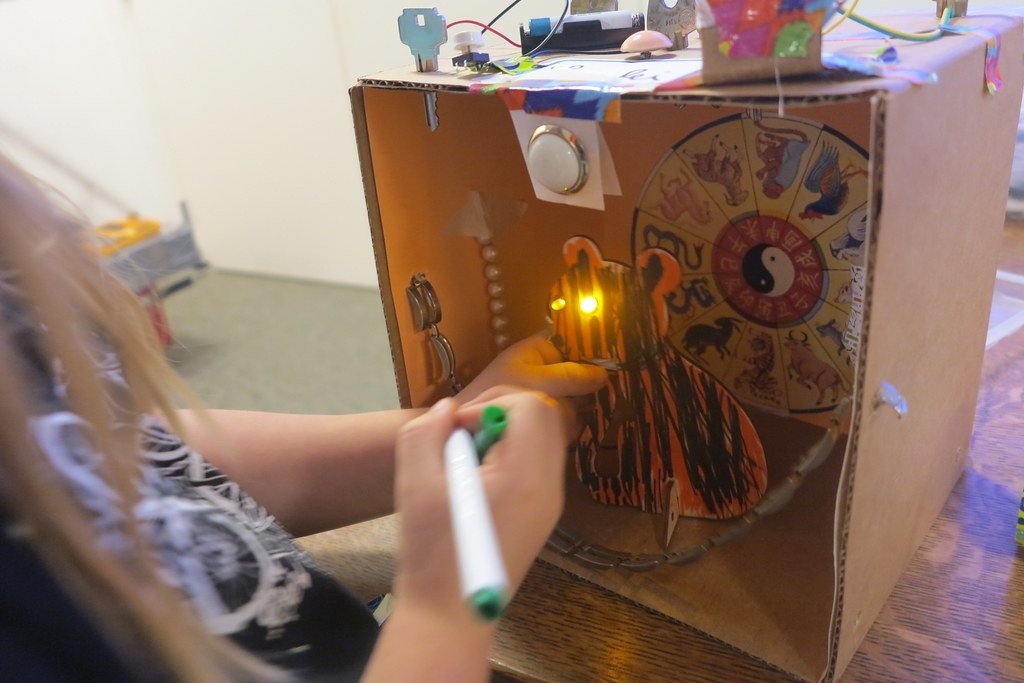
[0,143,611,683]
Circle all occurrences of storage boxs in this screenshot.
[344,0,1024,682]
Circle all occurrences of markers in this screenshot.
[441,426,514,623]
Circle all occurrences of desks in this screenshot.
[374,211,1024,683]
